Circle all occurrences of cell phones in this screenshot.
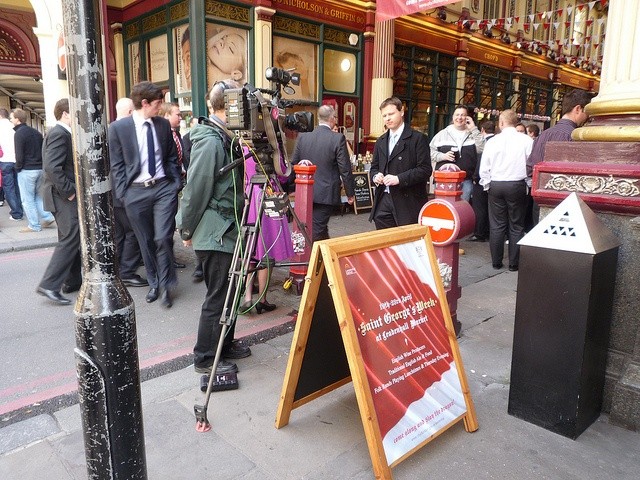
[465,117,469,124]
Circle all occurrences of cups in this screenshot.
[450,146,459,163]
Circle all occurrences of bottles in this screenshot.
[358,161,364,172]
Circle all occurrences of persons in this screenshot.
[288,105,356,241]
[230,129,294,315]
[36,98,80,304]
[426,105,486,255]
[368,97,432,229]
[208,26,246,86]
[526,123,542,141]
[0,106,27,228]
[329,120,345,138]
[513,121,528,135]
[181,112,200,168]
[176,30,196,97]
[11,112,55,234]
[478,109,536,272]
[158,104,187,182]
[108,83,181,309]
[175,84,251,372]
[527,90,592,192]
[278,53,316,100]
[114,94,137,120]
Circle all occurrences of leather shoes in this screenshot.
[466,234,486,242]
[192,269,203,277]
[493,264,503,269]
[509,264,518,271]
[146,288,158,303]
[19,227,39,233]
[37,285,71,304]
[62,283,80,293]
[121,275,149,287]
[161,290,171,307]
[172,261,185,268]
[40,220,54,228]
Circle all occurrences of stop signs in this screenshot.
[58,33,67,71]
[418,200,459,244]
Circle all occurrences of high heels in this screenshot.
[256,300,276,314]
[239,300,251,313]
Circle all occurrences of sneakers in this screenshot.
[9,216,21,221]
[222,343,251,359]
[195,360,238,376]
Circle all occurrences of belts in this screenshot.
[130,177,167,188]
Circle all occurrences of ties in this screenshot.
[389,134,397,156]
[144,122,156,178]
[172,128,183,168]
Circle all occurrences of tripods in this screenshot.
[194,169,317,431]
